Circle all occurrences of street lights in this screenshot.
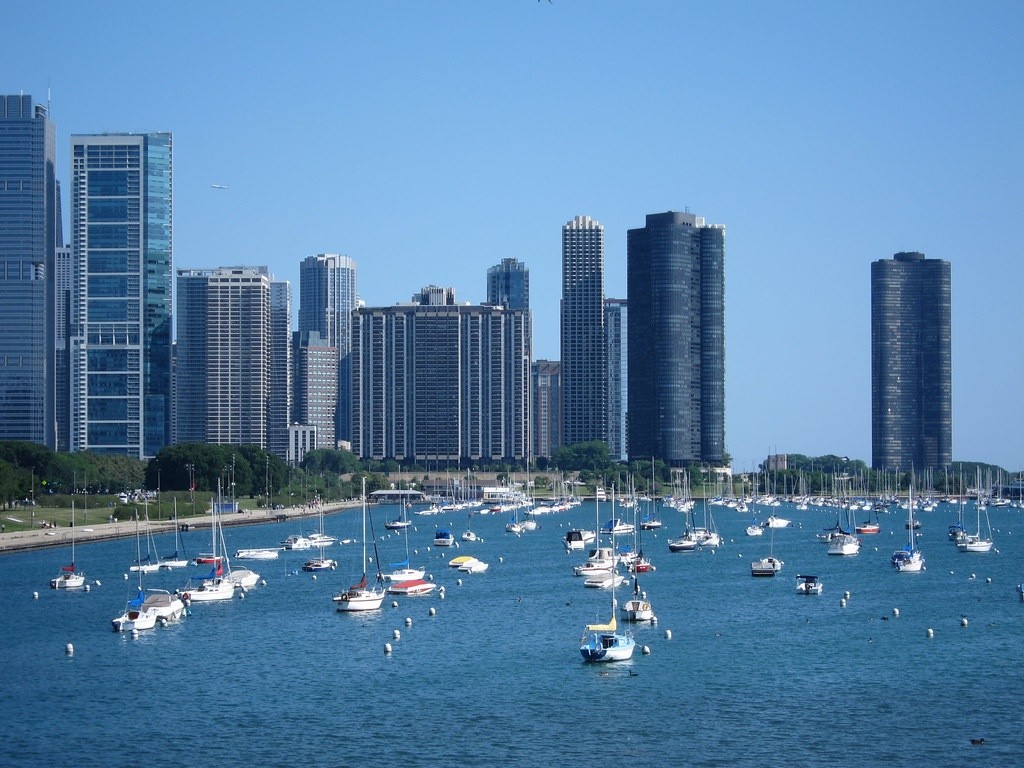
[224,465,233,498]
[266,455,270,510]
[233,453,236,513]
[186,463,194,503]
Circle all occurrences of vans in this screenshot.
[119,494,128,504]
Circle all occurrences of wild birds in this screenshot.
[566,601,572,606]
[629,671,639,676]
[868,637,872,642]
[806,619,809,624]
[969,738,984,744]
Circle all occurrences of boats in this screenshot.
[796,574,823,594]
[306,529,338,547]
[234,547,285,560]
[144,587,185,627]
[386,579,436,595]
[280,534,312,550]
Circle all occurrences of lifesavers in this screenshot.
[342,594,347,600]
[183,594,188,599]
[595,642,603,652]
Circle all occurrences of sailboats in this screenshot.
[580,481,636,662]
[302,498,334,572]
[383,459,1024,589]
[160,496,189,567]
[336,476,386,611]
[620,499,654,621]
[751,481,782,576]
[178,476,261,603]
[376,498,426,582]
[130,498,161,572]
[110,505,159,631]
[50,500,86,588]
